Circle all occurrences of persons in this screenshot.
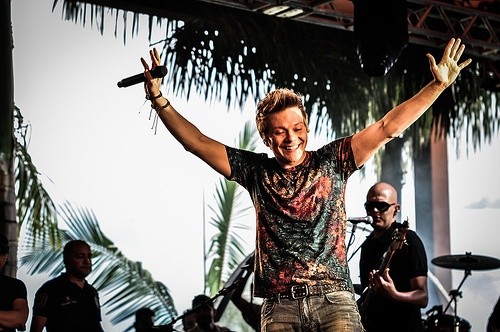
[141,38,472,332]
[0,233,29,332]
[359,182,428,332]
[29,240,105,332]
[134,289,262,332]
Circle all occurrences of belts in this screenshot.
[264,284,353,300]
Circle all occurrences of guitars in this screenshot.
[359,216,409,326]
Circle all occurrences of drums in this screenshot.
[427,314,471,332]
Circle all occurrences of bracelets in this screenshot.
[139,91,162,113]
[149,99,170,135]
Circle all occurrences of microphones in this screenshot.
[117,66,168,88]
[220,277,246,295]
[348,215,373,224]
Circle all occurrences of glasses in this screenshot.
[365,202,395,212]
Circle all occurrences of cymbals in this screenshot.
[431,254,500,270]
